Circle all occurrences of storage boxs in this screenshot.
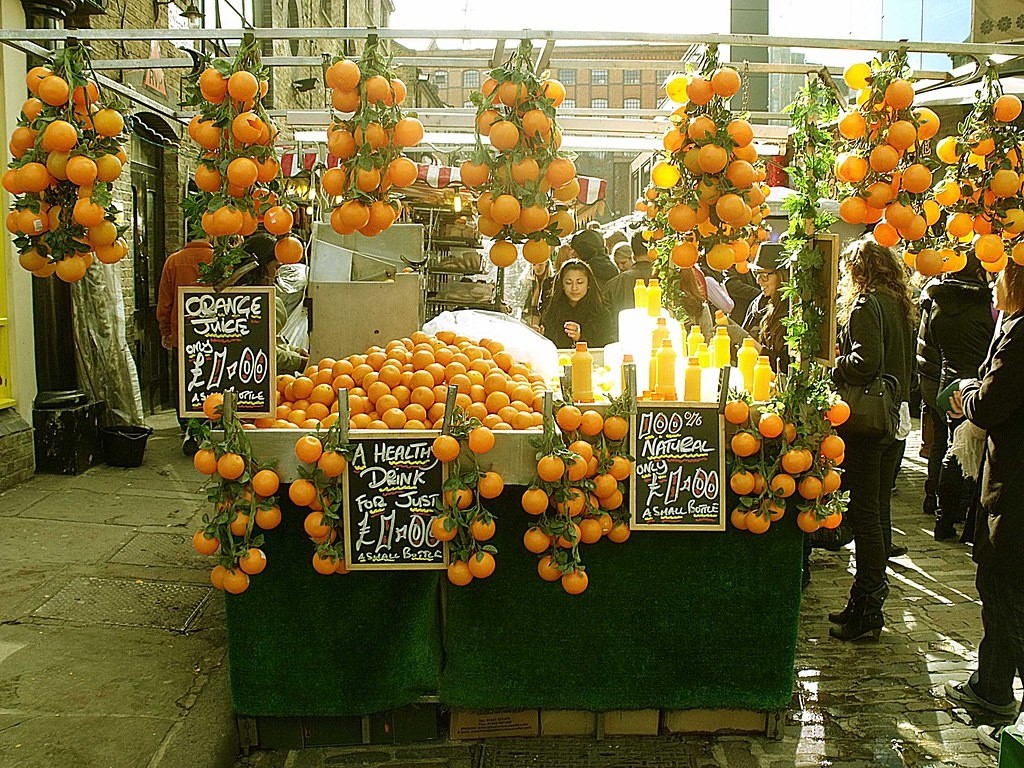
[450,708,768,741]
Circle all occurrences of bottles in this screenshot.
[621,355,634,395]
[715,309,729,327]
[647,278,661,316]
[648,318,713,401]
[571,342,593,401]
[714,327,730,368]
[634,279,647,308]
[753,356,771,401]
[736,338,759,394]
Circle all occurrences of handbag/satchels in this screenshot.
[832,292,900,444]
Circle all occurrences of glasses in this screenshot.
[752,271,777,282]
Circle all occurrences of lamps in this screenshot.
[153,0,206,23]
[417,74,431,82]
[292,78,320,92]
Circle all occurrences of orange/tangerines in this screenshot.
[432,427,503,586]
[460,78,580,268]
[835,62,1023,276]
[521,406,630,594]
[193,393,282,595]
[724,399,852,533]
[321,59,424,237]
[240,329,553,431]
[634,68,771,272]
[187,69,303,265]
[0,66,128,282]
[289,436,346,575]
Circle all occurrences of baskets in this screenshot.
[102,424,151,468]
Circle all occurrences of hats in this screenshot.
[571,231,604,259]
[747,242,787,270]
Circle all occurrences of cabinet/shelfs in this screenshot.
[422,208,511,321]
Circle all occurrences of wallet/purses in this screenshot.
[937,379,962,416]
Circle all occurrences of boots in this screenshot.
[934,508,957,540]
[920,412,934,458]
[829,580,860,624]
[924,479,938,514]
[830,582,889,640]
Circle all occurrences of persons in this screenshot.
[909,244,998,542]
[944,256,1024,752]
[520,220,792,376]
[153,215,310,458]
[828,232,919,639]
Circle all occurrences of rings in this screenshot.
[577,326,580,333]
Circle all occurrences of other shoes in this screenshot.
[802,570,811,589]
[183,426,198,455]
[891,545,908,557]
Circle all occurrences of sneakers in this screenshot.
[944,680,1014,714]
[977,725,1002,752]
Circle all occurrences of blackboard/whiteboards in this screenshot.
[629,401,726,532]
[341,429,450,570]
[787,234,839,369]
[177,285,277,419]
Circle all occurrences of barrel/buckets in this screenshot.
[102,424,153,467]
